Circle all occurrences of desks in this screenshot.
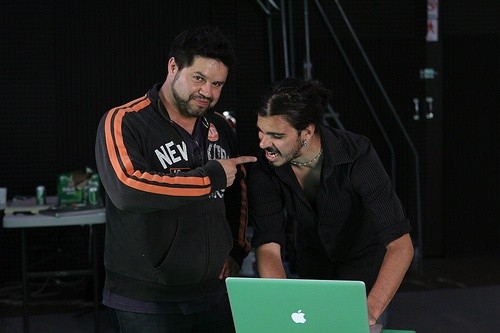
[3,198,106,333]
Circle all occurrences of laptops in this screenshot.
[225,277,416,333]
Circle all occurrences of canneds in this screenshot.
[35,186,47,206]
[80,174,101,205]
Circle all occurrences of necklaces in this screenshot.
[290,148,322,166]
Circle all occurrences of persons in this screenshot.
[250,74,416,329]
[93,24,258,333]
